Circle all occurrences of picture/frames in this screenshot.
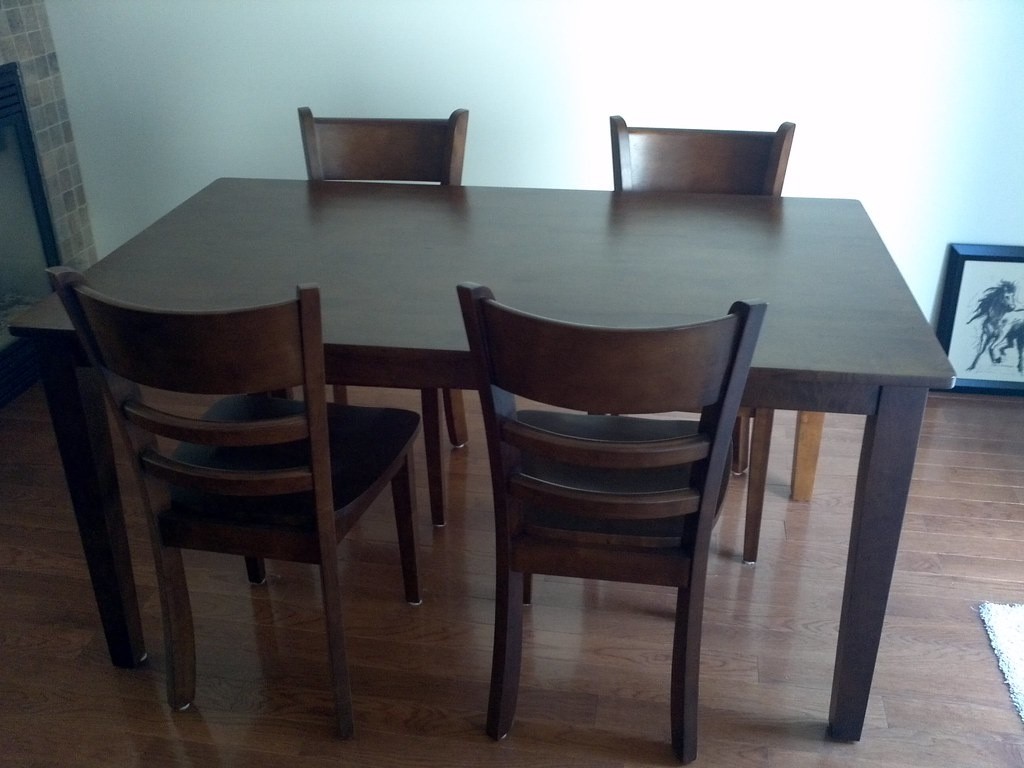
[930,243,1024,396]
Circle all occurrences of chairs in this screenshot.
[455,282,767,764]
[46,266,425,739]
[611,116,795,563]
[298,107,469,527]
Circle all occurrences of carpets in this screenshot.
[979,601,1024,724]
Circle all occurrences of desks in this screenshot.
[9,177,956,744]
